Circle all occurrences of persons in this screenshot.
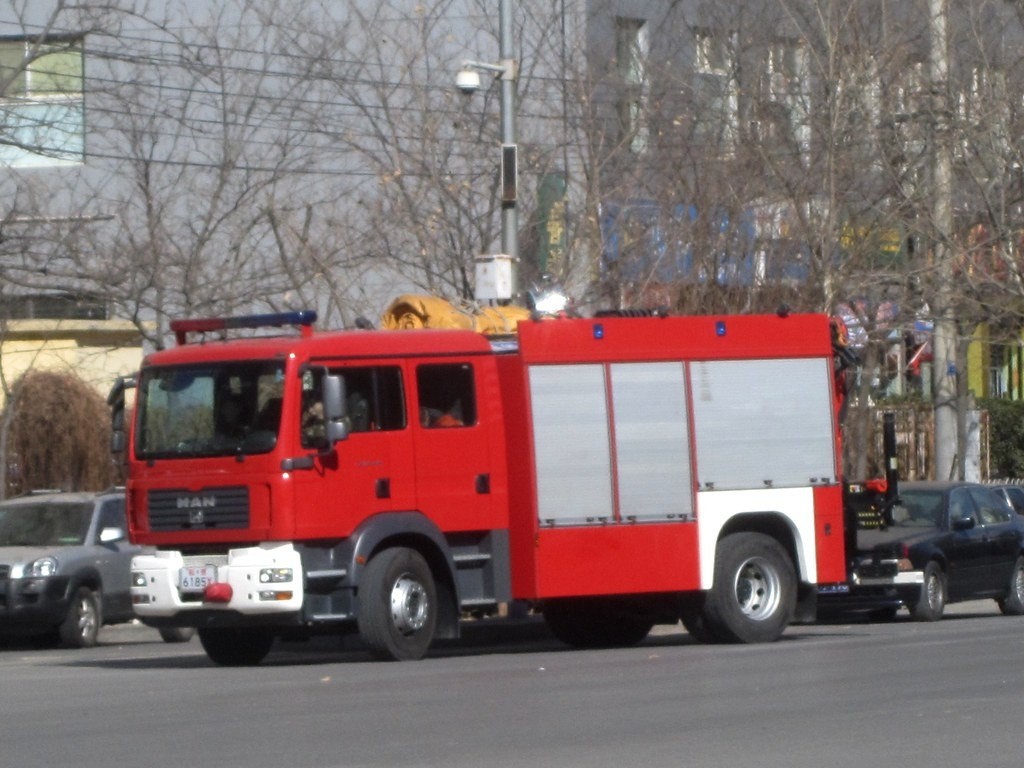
[301,390,352,440]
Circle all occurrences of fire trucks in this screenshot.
[107,309,852,665]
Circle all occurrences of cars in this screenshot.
[983,483,1024,516]
[816,480,1024,622]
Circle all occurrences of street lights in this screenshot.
[453,59,521,309]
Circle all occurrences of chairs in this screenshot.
[347,388,371,434]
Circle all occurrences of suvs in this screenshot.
[0,485,196,650]
